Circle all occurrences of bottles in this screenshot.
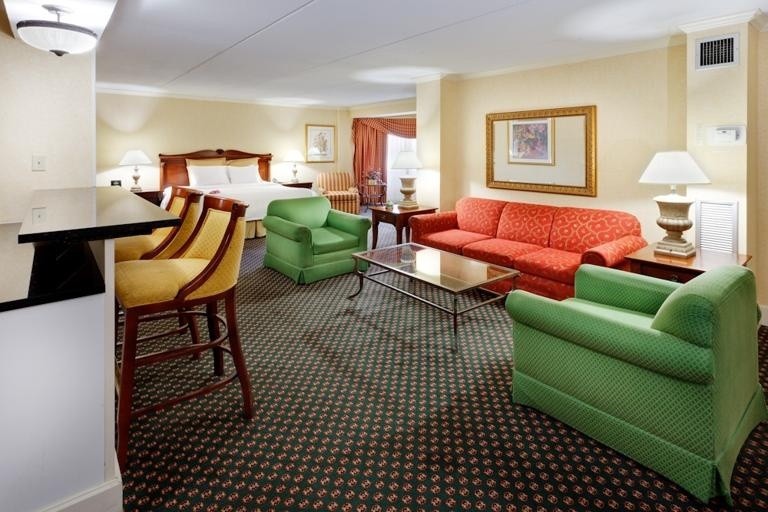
[386,200,393,208]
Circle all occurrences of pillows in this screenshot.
[185,156,263,186]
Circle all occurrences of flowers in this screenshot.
[362,169,384,184]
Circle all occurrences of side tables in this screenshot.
[367,203,439,267]
[359,181,388,213]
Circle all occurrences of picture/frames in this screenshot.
[506,117,556,167]
[485,104,598,198]
[304,123,337,164]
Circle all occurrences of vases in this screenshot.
[367,179,377,185]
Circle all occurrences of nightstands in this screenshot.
[134,190,160,205]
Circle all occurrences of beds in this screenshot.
[158,148,318,240]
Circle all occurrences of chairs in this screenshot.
[115,184,258,475]
[316,171,360,215]
[114,184,204,361]
[504,262,768,509]
[261,195,372,286]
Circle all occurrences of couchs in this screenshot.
[407,196,648,301]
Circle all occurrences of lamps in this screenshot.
[14,4,100,58]
[118,150,153,192]
[282,150,305,183]
[636,150,715,260]
[389,150,425,210]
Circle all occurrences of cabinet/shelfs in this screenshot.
[623,239,753,284]
